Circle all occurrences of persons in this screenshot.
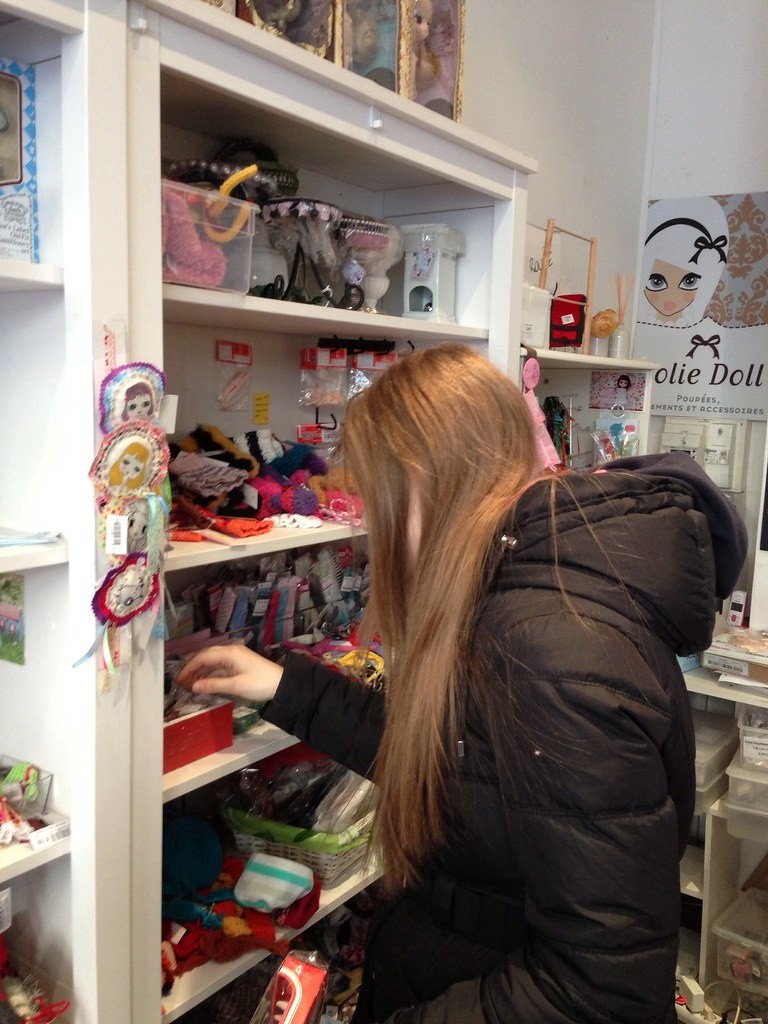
[173,342,752,1024]
[409,0,442,101]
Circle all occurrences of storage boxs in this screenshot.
[247,951,328,1024]
[725,748,768,811]
[737,701,768,773]
[712,887,767,998]
[202,0,466,123]
[693,767,728,816]
[688,705,738,785]
[159,179,261,297]
[0,753,53,824]
[0,952,76,1024]
[164,693,233,774]
[520,283,551,347]
[229,606,304,672]
[723,794,767,844]
[700,633,768,684]
[275,593,360,647]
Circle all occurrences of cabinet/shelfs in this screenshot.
[519,346,767,1023]
[124,0,540,1024]
[0,0,133,1024]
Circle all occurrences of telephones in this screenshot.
[726,590,748,629]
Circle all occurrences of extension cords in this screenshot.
[674,993,721,1024]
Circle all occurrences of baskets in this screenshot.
[217,791,378,884]
